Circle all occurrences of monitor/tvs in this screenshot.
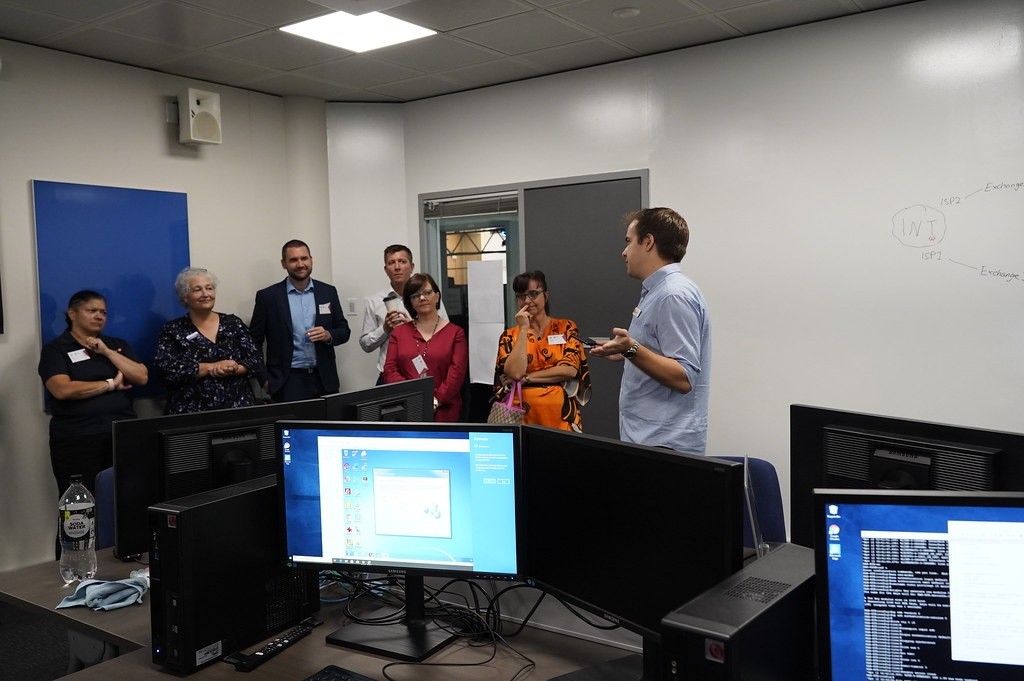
[111,376,1024,681]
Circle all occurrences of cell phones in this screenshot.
[572,334,599,345]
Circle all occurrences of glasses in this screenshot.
[409,289,434,301]
[515,290,542,302]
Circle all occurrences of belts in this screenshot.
[289,368,318,374]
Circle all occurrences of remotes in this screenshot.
[235,624,312,673]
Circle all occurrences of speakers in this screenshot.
[179,89,223,145]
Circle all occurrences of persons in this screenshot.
[383,273,468,422]
[359,245,450,386]
[248,239,352,403]
[588,207,713,456]
[149,267,263,416]
[37,290,148,561]
[492,271,592,433]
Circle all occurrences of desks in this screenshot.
[0,546,640,681]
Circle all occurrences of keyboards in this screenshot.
[302,664,377,681]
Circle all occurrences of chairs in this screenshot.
[707,455,788,550]
[96,467,119,547]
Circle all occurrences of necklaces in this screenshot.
[415,314,440,359]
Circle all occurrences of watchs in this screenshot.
[623,342,639,359]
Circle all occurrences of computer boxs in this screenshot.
[146,474,320,674]
[662,541,819,681]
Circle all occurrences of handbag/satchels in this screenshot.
[487,379,527,426]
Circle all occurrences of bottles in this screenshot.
[304,326,314,359]
[58,475,97,583]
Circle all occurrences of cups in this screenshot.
[382,296,401,314]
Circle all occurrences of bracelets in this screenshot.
[524,374,531,384]
[106,379,115,391]
